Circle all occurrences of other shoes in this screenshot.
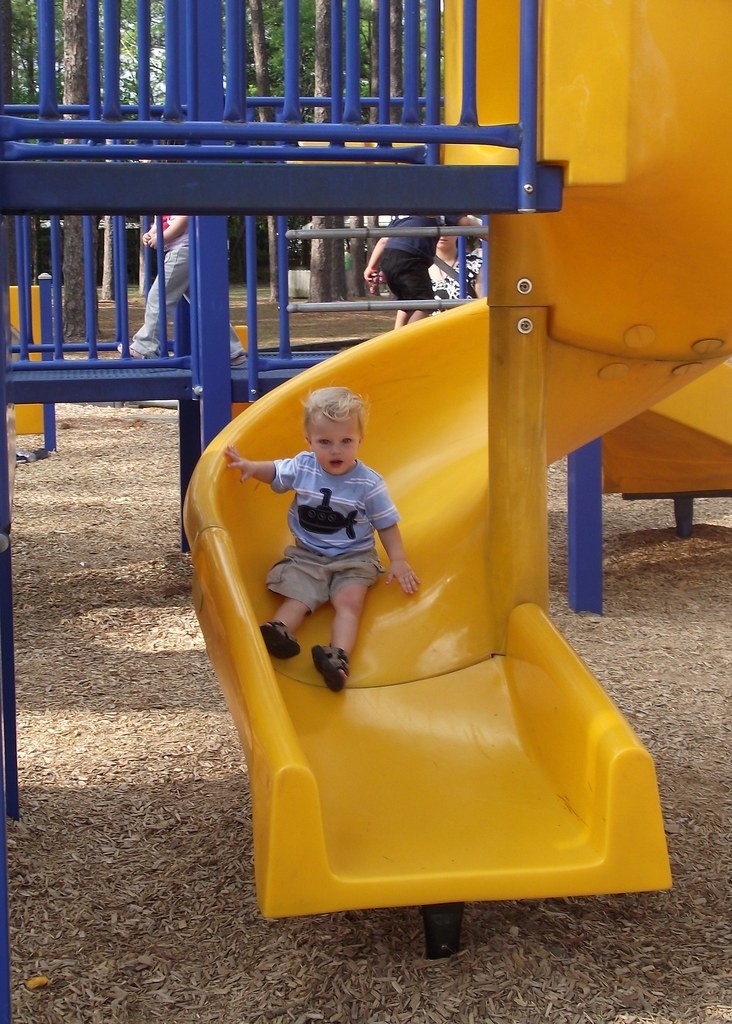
[229,354,248,367]
[116,342,163,362]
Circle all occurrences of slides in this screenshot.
[182,295,675,921]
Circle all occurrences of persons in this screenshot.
[118,158,247,362]
[363,213,488,331]
[224,387,418,692]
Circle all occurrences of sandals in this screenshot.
[311,643,353,694]
[258,620,302,660]
